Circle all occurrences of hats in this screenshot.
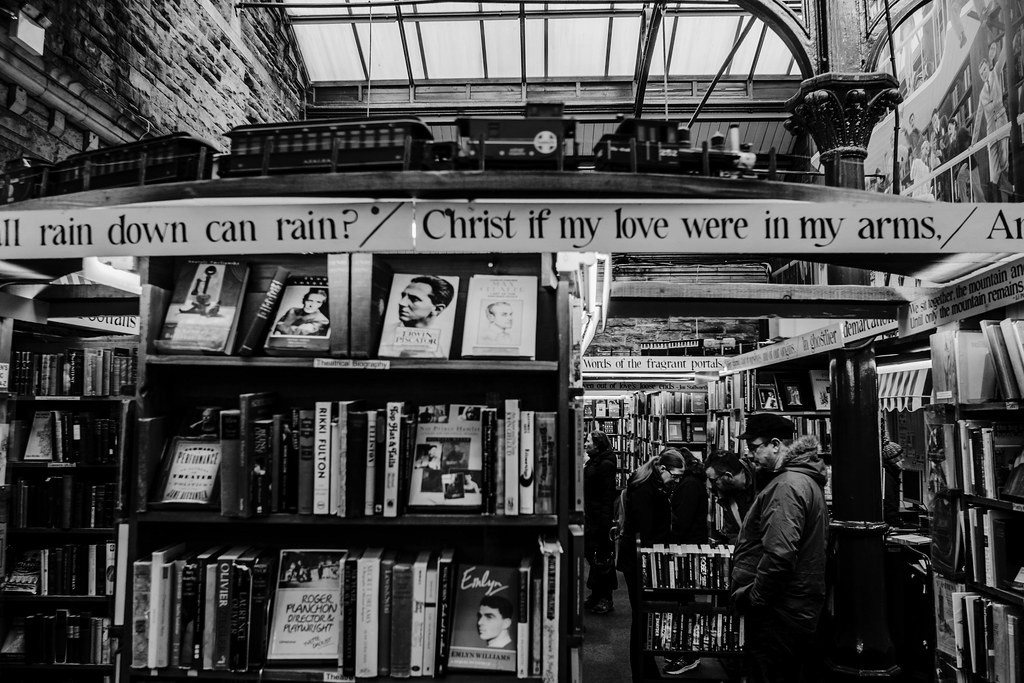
[736,413,794,441]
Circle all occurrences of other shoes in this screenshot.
[591,599,615,616]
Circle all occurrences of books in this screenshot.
[0,258,1024,683]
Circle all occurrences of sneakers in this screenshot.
[662,653,701,674]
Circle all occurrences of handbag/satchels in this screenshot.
[589,551,616,578]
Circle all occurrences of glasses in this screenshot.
[661,469,685,478]
[706,471,735,485]
[743,438,784,452]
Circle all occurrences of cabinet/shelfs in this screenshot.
[0,254,1024,683]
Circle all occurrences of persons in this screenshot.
[616,450,685,683]
[702,450,774,546]
[870,35,1024,204]
[479,303,515,346]
[881,438,905,528]
[185,265,220,316]
[582,431,616,615]
[382,276,454,351]
[478,594,515,648]
[414,444,439,480]
[659,447,709,675]
[462,475,480,494]
[273,287,330,337]
[441,441,468,468]
[730,413,830,683]
